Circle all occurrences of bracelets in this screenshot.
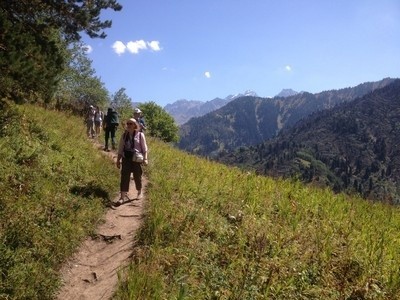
[144,159,148,160]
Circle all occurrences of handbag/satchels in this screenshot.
[132,151,143,163]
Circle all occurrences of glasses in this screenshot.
[126,123,133,125]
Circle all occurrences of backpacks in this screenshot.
[107,110,119,126]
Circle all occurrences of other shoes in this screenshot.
[112,145,115,150]
[116,199,125,205]
[137,193,143,200]
[103,147,108,151]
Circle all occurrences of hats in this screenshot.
[129,118,139,130]
[89,105,94,109]
[134,108,142,114]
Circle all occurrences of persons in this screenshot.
[84,105,146,151]
[117,118,148,205]
